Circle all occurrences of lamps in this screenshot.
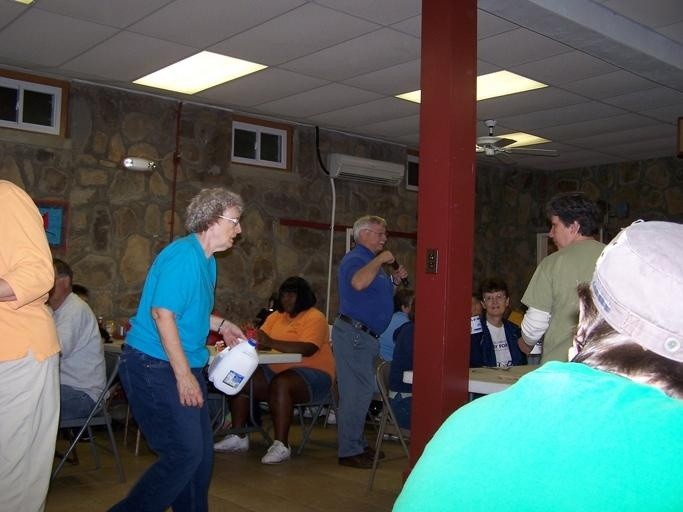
[117,155,157,173]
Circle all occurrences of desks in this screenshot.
[399,361,539,406]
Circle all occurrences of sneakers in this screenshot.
[291,405,337,426]
[260,440,291,464]
[69,427,96,443]
[213,432,249,453]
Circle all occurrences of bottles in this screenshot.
[97,316,127,336]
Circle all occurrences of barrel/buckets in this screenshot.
[207,337,260,396]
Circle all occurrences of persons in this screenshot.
[517,190,608,364]
[378,276,542,431]
[330,216,407,471]
[388,220,682,511]
[102,183,247,511]
[0,182,62,511]
[45,258,109,421]
[69,282,111,442]
[213,275,336,465]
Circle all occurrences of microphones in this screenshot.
[388,259,409,287]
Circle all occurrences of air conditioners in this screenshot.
[323,153,404,190]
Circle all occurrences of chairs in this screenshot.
[48,335,412,493]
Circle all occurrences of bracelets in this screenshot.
[216,319,225,334]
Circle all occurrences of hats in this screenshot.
[588,219,682,362]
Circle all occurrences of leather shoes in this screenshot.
[338,445,385,469]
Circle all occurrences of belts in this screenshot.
[339,314,379,339]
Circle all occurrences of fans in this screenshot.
[474,119,560,168]
